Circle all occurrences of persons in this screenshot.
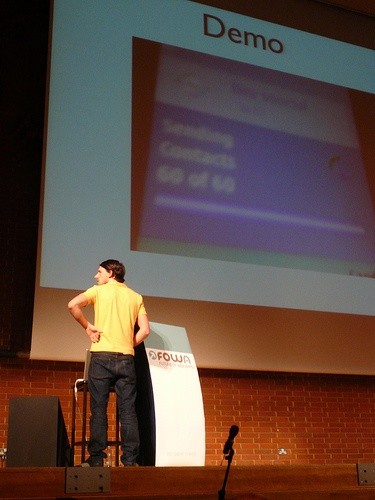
[67,258,150,468]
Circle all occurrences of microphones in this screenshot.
[223,425,239,454]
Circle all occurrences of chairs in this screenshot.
[71,349,122,467]
